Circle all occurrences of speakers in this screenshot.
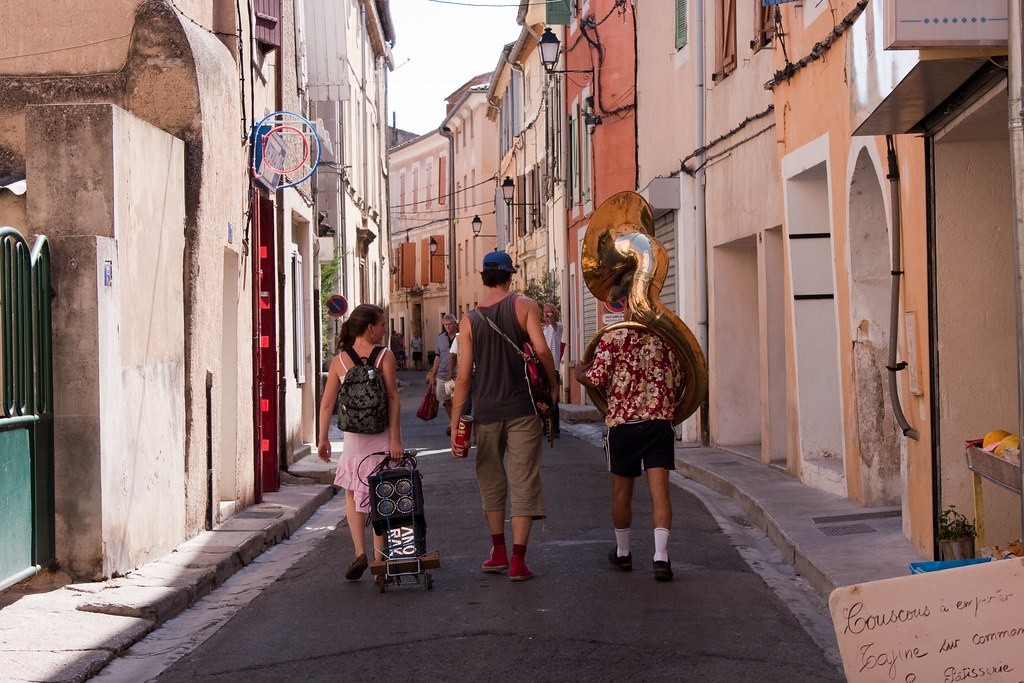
[367,469,427,536]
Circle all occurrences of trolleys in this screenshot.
[367,453,441,593]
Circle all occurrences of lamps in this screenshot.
[500,175,537,216]
[427,237,450,264]
[471,215,498,245]
[536,28,595,108]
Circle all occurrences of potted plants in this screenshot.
[939,504,978,562]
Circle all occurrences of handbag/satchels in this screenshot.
[523,341,542,385]
[416,385,439,421]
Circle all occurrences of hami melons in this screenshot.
[984,429,1020,458]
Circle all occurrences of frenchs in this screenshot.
[581,190,707,427]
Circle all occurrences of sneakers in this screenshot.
[652,557,674,578]
[509,555,535,580]
[608,544,632,571]
[482,545,509,570]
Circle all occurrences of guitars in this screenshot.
[523,341,553,449]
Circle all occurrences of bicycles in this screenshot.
[397,347,406,371]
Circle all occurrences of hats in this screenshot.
[483,252,517,274]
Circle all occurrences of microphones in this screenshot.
[372,449,417,456]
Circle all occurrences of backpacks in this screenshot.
[336,346,389,434]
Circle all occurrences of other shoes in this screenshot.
[345,554,368,580]
[446,426,451,436]
[376,575,393,586]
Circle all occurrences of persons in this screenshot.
[449,251,559,581]
[537,302,564,439]
[574,287,686,582]
[318,304,405,585]
[410,331,424,371]
[426,312,478,445]
[390,330,408,371]
